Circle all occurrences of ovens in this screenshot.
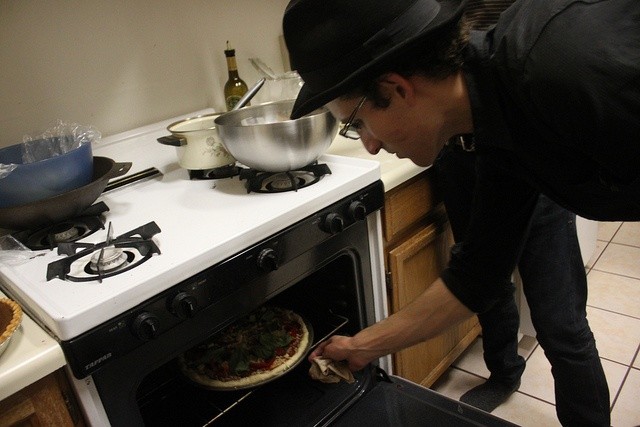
[74,209,527,426]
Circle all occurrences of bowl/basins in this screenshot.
[0,134,94,209]
[213,98,339,173]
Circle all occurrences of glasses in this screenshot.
[339,95,367,139]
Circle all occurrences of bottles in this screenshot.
[223,48,251,111]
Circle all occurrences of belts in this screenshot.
[450,135,476,152]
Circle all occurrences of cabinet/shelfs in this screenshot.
[1,372,75,427]
[382,166,483,389]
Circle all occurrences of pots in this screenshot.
[157,112,237,171]
[0,156,133,229]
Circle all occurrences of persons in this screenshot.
[433,126,611,427]
[284,0,639,374]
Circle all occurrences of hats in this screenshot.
[283,0,464,120]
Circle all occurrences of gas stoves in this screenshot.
[0,107,382,380]
